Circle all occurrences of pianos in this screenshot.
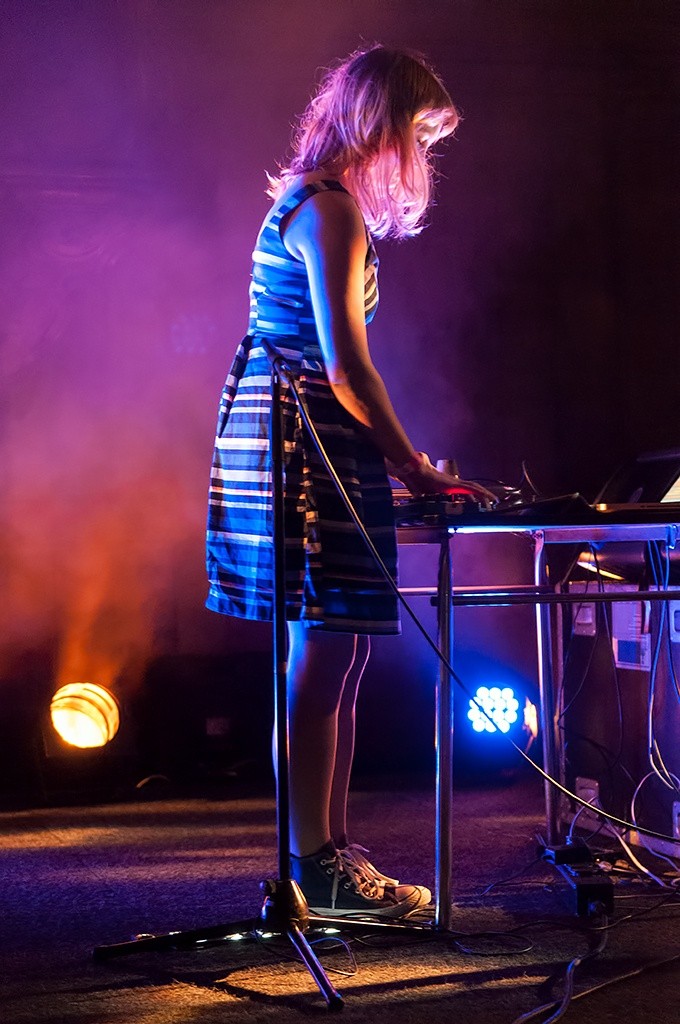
[393,490,680,931]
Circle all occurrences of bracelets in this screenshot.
[388,452,431,480]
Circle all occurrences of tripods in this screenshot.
[92,351,443,1014]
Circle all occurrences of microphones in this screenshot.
[260,336,294,385]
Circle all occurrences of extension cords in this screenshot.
[556,861,615,916]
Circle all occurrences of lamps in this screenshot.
[463,681,539,781]
[31,680,124,806]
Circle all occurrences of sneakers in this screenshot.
[338,842,432,907]
[290,837,422,918]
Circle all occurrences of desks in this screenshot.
[397,523,680,930]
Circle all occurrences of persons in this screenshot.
[203,47,499,923]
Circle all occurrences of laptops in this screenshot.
[595,449,680,505]
